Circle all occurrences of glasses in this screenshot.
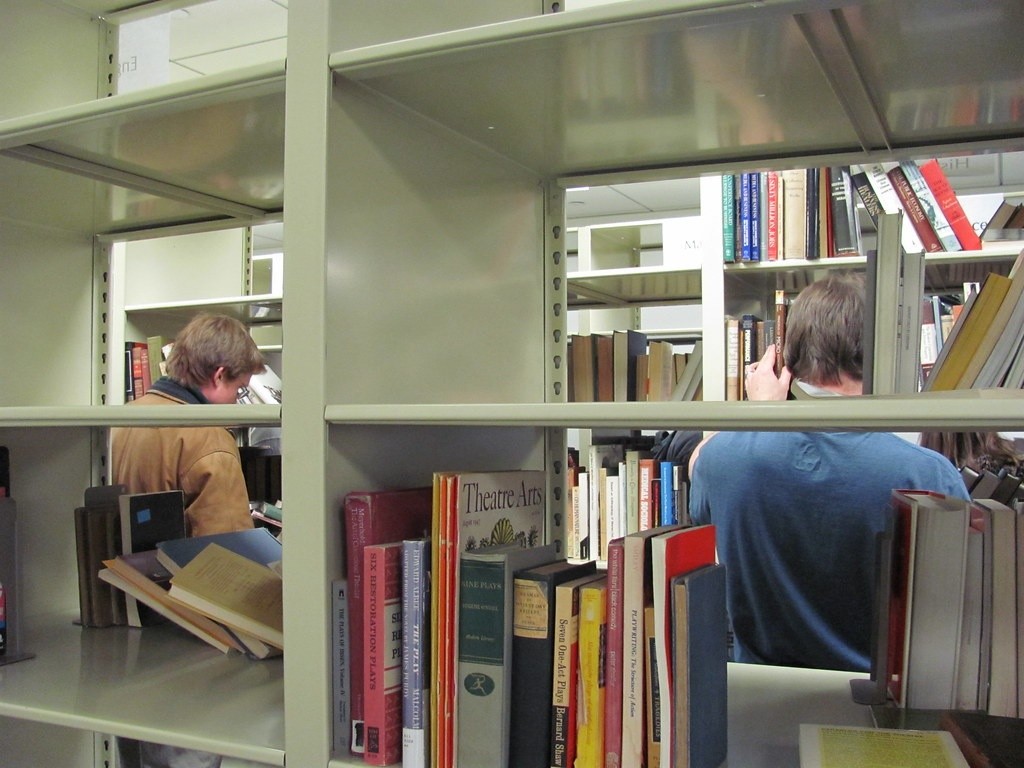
[236,374,249,399]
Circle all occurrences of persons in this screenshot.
[248,426,281,506]
[917,429,1021,471]
[111,312,265,537]
[687,279,971,673]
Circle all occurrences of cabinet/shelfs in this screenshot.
[0,0,1024,768]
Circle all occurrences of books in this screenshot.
[125,336,282,405]
[863,208,1023,394]
[723,157,1023,263]
[332,470,728,768]
[568,441,686,562]
[799,488,1024,767]
[73,484,282,658]
[725,290,796,400]
[568,330,702,402]
